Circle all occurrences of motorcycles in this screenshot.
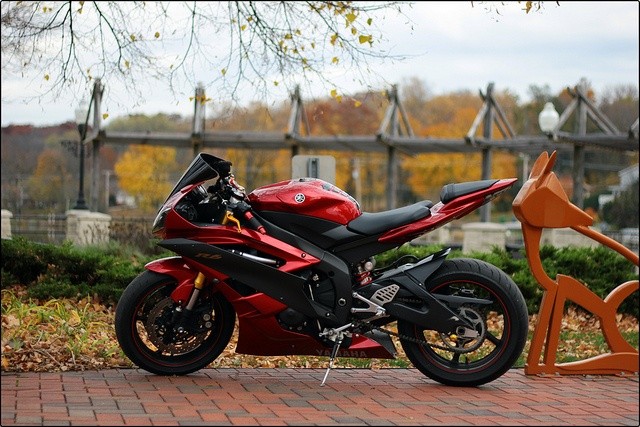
[115,153,528,388]
